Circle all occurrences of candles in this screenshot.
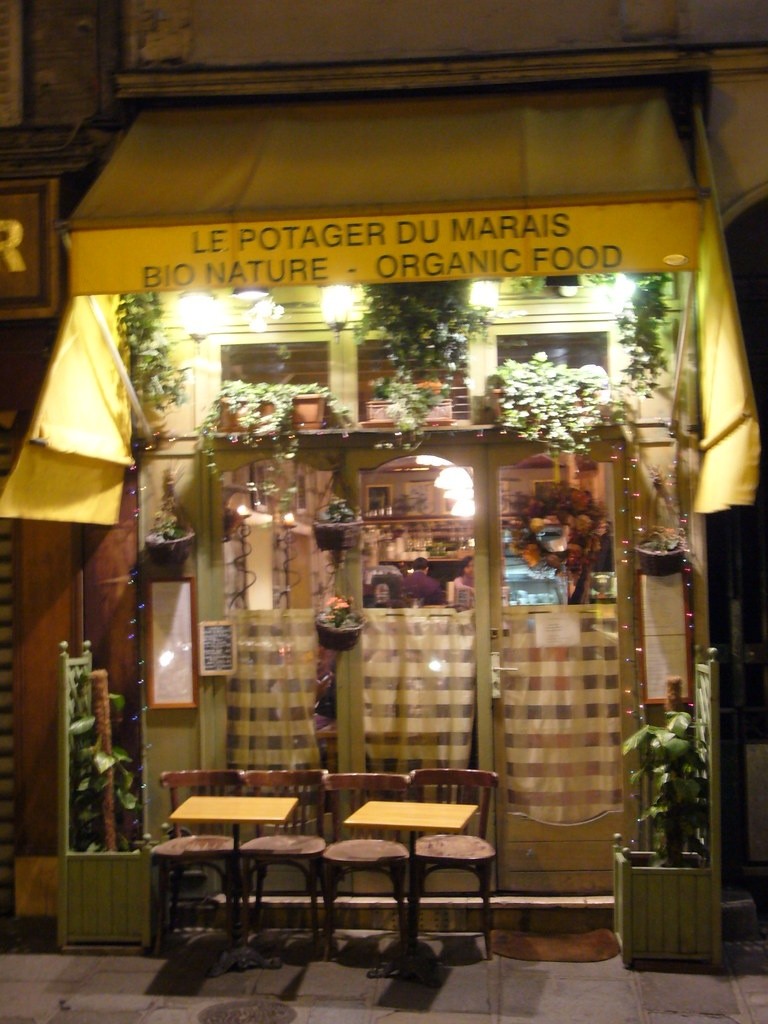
[611,646,721,969]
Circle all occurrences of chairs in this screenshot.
[410,770,499,967]
[244,770,328,953]
[323,773,407,965]
[149,769,242,955]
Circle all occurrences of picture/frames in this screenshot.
[365,483,393,516]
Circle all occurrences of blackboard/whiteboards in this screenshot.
[199,620,237,677]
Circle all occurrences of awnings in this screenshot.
[58,77,712,295]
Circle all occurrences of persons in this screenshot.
[453,556,475,612]
[405,557,443,605]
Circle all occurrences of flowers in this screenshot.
[512,481,609,573]
[316,589,365,627]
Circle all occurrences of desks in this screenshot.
[172,795,299,976]
[379,545,475,573]
[344,801,479,986]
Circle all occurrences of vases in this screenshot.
[313,620,362,652]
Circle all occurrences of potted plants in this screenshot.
[196,380,352,481]
[145,497,195,568]
[634,469,687,574]
[310,497,363,552]
[495,353,614,458]
[366,370,456,426]
[55,638,158,953]
